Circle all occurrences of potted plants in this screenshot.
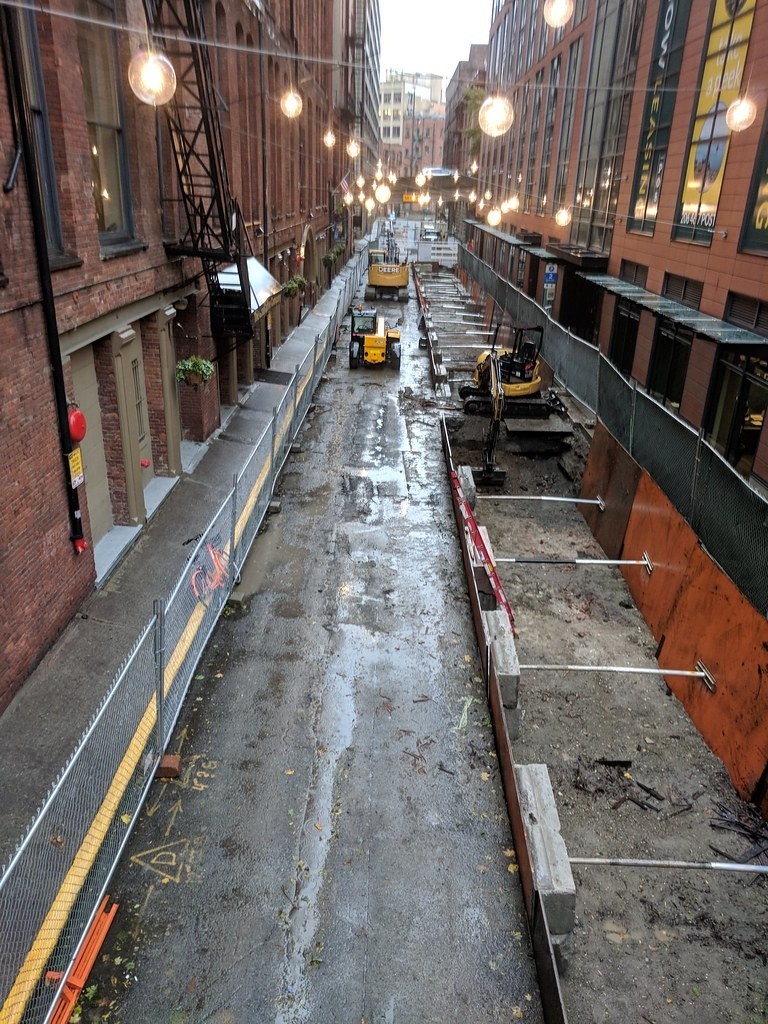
[283,275,308,296]
[175,353,214,389]
[322,244,346,266]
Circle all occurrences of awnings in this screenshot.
[209,256,286,326]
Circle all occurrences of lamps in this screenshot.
[478,94,514,138]
[725,97,757,132]
[128,50,177,107]
[280,91,303,118]
[556,210,571,227]
[543,0,574,27]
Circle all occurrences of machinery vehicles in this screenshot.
[348,305,401,373]
[363,218,411,304]
[458,323,551,419]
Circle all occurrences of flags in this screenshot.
[341,170,351,192]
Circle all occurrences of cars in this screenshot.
[418,230,442,243]
